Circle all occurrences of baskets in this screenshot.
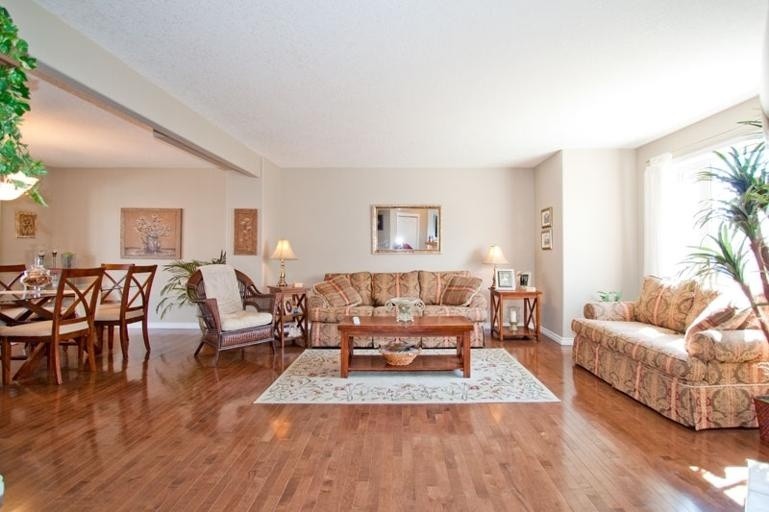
[378,345,421,365]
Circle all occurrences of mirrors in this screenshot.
[371,203,444,255]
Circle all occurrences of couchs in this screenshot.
[571,274,769,431]
[306,270,488,348]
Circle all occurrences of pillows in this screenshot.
[636,275,769,352]
[312,271,483,310]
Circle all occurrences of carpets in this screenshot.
[252,348,561,404]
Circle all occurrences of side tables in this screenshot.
[266,285,309,348]
[488,286,544,343]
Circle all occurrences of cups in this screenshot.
[519,274,530,285]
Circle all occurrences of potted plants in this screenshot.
[677,121,769,448]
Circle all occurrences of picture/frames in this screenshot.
[541,207,553,228]
[16,211,37,238]
[495,269,516,291]
[120,208,183,260]
[234,208,257,256]
[541,229,553,249]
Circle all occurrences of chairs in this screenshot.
[187,264,280,366]
[0,264,157,385]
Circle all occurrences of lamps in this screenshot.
[269,239,299,287]
[481,246,509,289]
[0,170,40,201]
